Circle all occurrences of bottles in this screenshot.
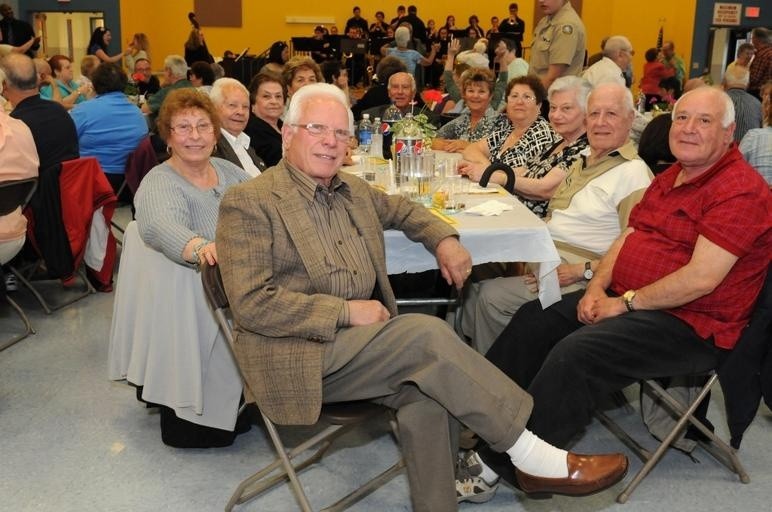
[356,100,423,190]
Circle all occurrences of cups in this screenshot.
[357,148,465,212]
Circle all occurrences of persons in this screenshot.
[133,0,771,361]
[451,81,772,505]
[1,28,162,281]
[212,78,632,512]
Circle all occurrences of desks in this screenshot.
[338,128,551,347]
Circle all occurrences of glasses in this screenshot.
[168,121,214,136]
[507,93,537,105]
[620,49,635,56]
[291,123,354,145]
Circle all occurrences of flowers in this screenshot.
[380,112,440,156]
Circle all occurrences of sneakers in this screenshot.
[21,265,59,281]
[389,418,479,450]
[455,449,502,504]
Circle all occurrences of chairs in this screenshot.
[0,133,157,356]
[201,258,410,512]
[587,257,771,504]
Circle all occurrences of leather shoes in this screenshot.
[515,451,629,499]
